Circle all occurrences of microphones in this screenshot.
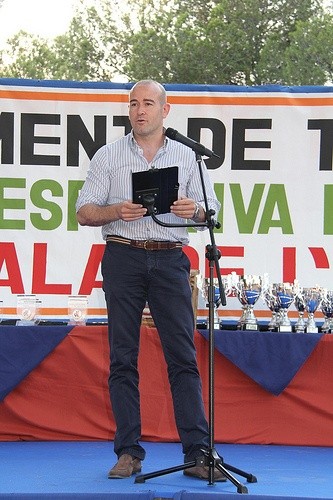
[165,128,221,160]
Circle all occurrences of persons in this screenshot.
[75,79,230,483]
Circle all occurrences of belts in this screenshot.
[106,236,182,251]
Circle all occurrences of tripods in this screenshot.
[135,151,258,494]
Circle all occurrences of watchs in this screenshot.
[193,202,199,219]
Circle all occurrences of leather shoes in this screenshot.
[108,452,142,478]
[184,466,227,481]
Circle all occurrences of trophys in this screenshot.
[200,274,333,334]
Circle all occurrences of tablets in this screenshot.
[132,166,179,216]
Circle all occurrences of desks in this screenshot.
[0,325,333,447]
